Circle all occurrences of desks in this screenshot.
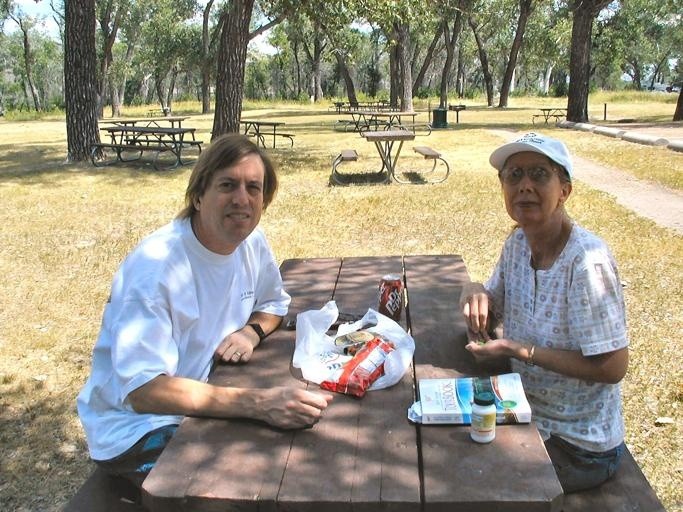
[140,251,566,512]
[538,108,567,123]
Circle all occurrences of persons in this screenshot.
[458,132,630,494]
[74,134,333,495]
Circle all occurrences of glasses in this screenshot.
[500,167,558,182]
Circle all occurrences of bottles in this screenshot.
[470,391,495,444]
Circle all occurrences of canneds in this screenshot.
[378,274,404,320]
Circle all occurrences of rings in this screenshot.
[234,350,244,358]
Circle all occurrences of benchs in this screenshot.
[558,434,666,511]
[327,99,450,188]
[58,465,145,511]
[532,113,566,123]
[544,115,567,125]
[88,116,298,172]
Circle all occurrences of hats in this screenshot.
[490,132,573,178]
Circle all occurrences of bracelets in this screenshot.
[244,322,267,346]
[525,346,539,366]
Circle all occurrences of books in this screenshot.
[419,374,532,426]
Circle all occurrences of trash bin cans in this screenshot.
[432,109,447,128]
